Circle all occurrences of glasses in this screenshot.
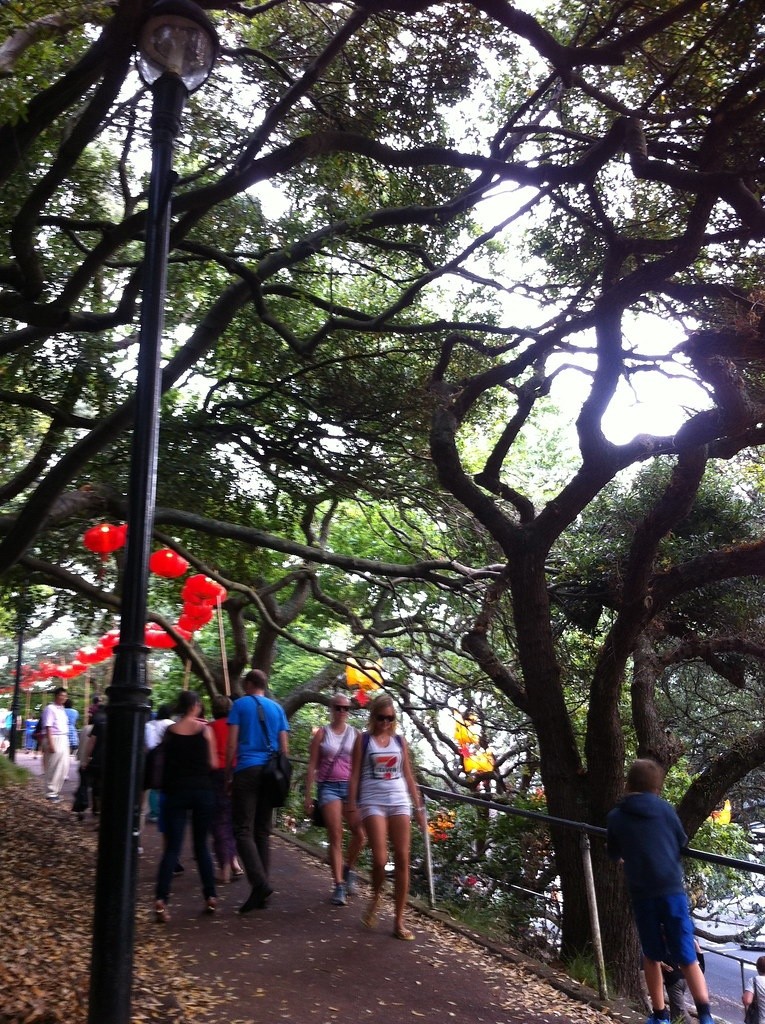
[335,705,351,712]
[373,713,395,722]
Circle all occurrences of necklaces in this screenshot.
[377,734,384,743]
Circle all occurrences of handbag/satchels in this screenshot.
[265,753,292,807]
[70,769,89,813]
[744,978,760,1024]
[312,800,327,828]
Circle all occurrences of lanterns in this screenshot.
[0,630,120,695]
[84,523,125,562]
[143,573,228,649]
[150,546,189,578]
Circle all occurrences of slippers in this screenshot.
[393,929,415,940]
[360,911,379,928]
[233,871,244,876]
[217,879,231,884]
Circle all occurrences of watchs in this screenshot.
[415,807,425,812]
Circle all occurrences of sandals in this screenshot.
[155,900,171,923]
[205,896,217,910]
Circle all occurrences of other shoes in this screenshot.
[257,897,269,909]
[171,863,185,874]
[239,884,273,914]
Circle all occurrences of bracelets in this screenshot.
[347,810,355,812]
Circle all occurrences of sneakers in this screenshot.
[343,866,357,893]
[646,1015,670,1024]
[331,884,347,904]
[700,1016,715,1024]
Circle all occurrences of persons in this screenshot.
[133,699,244,883]
[225,668,293,913]
[303,695,365,905]
[660,937,705,1024]
[346,695,428,940]
[30,687,109,816]
[742,956,765,1024]
[606,760,715,1024]
[25,712,39,753]
[151,691,218,923]
